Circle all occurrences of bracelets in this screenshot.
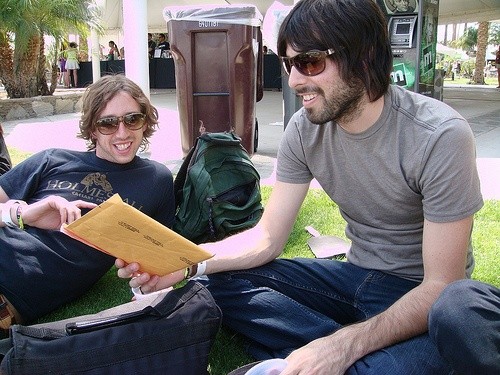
[184,260,207,281]
[2,199,29,229]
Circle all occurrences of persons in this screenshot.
[494,45,500,89]
[148,33,157,57]
[0,124,12,177]
[454,64,461,79]
[58,42,81,89]
[1,1,484,375]
[0,74,176,341]
[426,278,500,375]
[157,33,171,57]
[109,41,118,55]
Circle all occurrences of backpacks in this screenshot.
[171,131,264,240]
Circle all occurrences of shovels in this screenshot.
[304,226,350,258]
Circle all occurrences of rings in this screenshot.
[139,286,144,295]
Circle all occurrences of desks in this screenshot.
[71,58,176,89]
[263,53,282,92]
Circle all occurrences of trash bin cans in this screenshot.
[163,5,263,159]
[272,8,304,132]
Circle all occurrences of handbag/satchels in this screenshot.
[0,280,223,375]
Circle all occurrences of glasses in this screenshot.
[280,45,346,77]
[95,112,147,135]
